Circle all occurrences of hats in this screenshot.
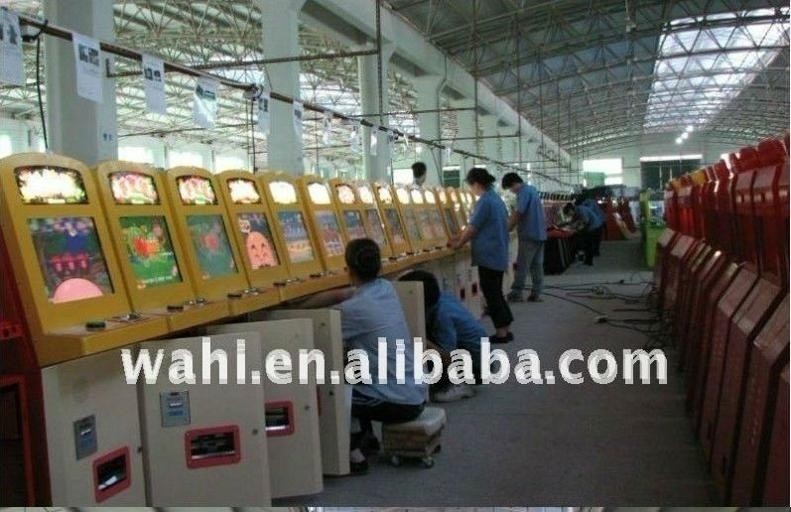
[464,168,489,183]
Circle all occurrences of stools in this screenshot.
[381,407,446,468]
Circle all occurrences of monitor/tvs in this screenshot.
[26,201,475,304]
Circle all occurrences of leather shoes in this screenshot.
[350,436,381,473]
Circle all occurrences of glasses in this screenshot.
[343,265,350,271]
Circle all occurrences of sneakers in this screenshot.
[489,331,514,343]
[504,291,545,303]
[432,383,475,402]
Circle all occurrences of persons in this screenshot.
[398,269,491,402]
[411,161,427,185]
[563,194,602,266]
[299,238,428,472]
[502,173,546,302]
[452,168,514,344]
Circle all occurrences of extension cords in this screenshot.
[595,315,609,324]
